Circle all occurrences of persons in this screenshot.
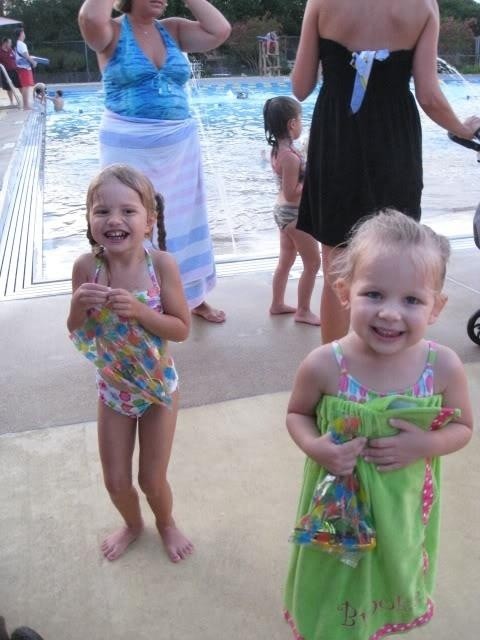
[77,0,232,323]
[35,88,47,105]
[0,36,23,109]
[285,208,474,640]
[262,97,322,326]
[66,164,194,562]
[15,27,37,110]
[290,1,480,345]
[44,90,66,113]
